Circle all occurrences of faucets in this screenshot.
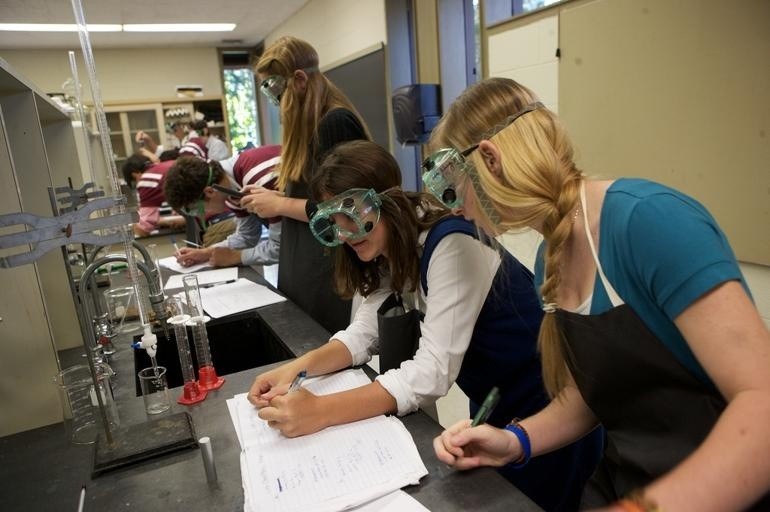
[84,238,160,335]
[79,254,167,365]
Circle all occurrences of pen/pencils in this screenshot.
[288,369,307,393]
[77,483,86,512]
[204,279,235,288]
[182,239,204,248]
[446,386,501,469]
[170,235,186,265]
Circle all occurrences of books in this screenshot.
[225,368,429,511]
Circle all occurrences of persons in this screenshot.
[196,122,230,161]
[246,141,605,498]
[162,145,289,267]
[121,153,194,237]
[425,76,770,511]
[135,121,208,161]
[238,36,375,336]
[157,137,208,160]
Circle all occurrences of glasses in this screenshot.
[421,149,474,209]
[259,74,286,106]
[179,199,204,220]
[309,188,382,248]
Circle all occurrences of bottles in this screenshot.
[166,297,203,401]
[183,274,218,388]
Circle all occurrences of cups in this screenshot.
[103,284,143,333]
[139,366,174,417]
[53,362,121,449]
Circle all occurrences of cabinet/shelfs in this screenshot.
[89,98,167,199]
[161,93,232,158]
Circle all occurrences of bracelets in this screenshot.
[502,417,531,468]
[601,486,659,511]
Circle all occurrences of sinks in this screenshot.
[132,306,298,399]
